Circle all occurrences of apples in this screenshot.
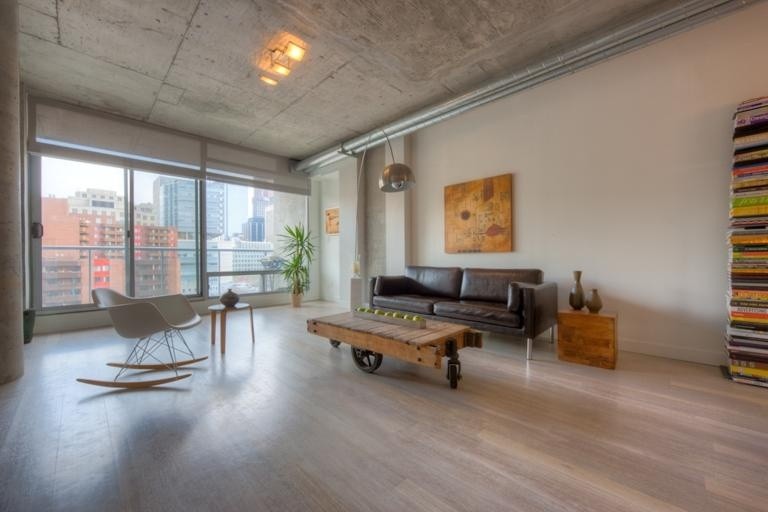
[357,307,421,321]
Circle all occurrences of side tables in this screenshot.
[208,302,255,353]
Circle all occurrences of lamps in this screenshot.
[351,129,417,277]
[259,42,306,85]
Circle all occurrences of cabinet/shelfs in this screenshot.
[557,308,619,369]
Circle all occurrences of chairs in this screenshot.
[76,288,208,388]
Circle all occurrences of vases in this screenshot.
[568,271,603,314]
[219,289,240,307]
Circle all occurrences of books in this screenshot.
[722,93,768,389]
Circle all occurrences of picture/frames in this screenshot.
[324,207,340,236]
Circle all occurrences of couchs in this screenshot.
[368,265,558,360]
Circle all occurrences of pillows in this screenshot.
[374,275,408,296]
[507,282,536,313]
[459,268,544,304]
[405,266,462,299]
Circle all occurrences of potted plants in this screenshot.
[281,222,314,308]
